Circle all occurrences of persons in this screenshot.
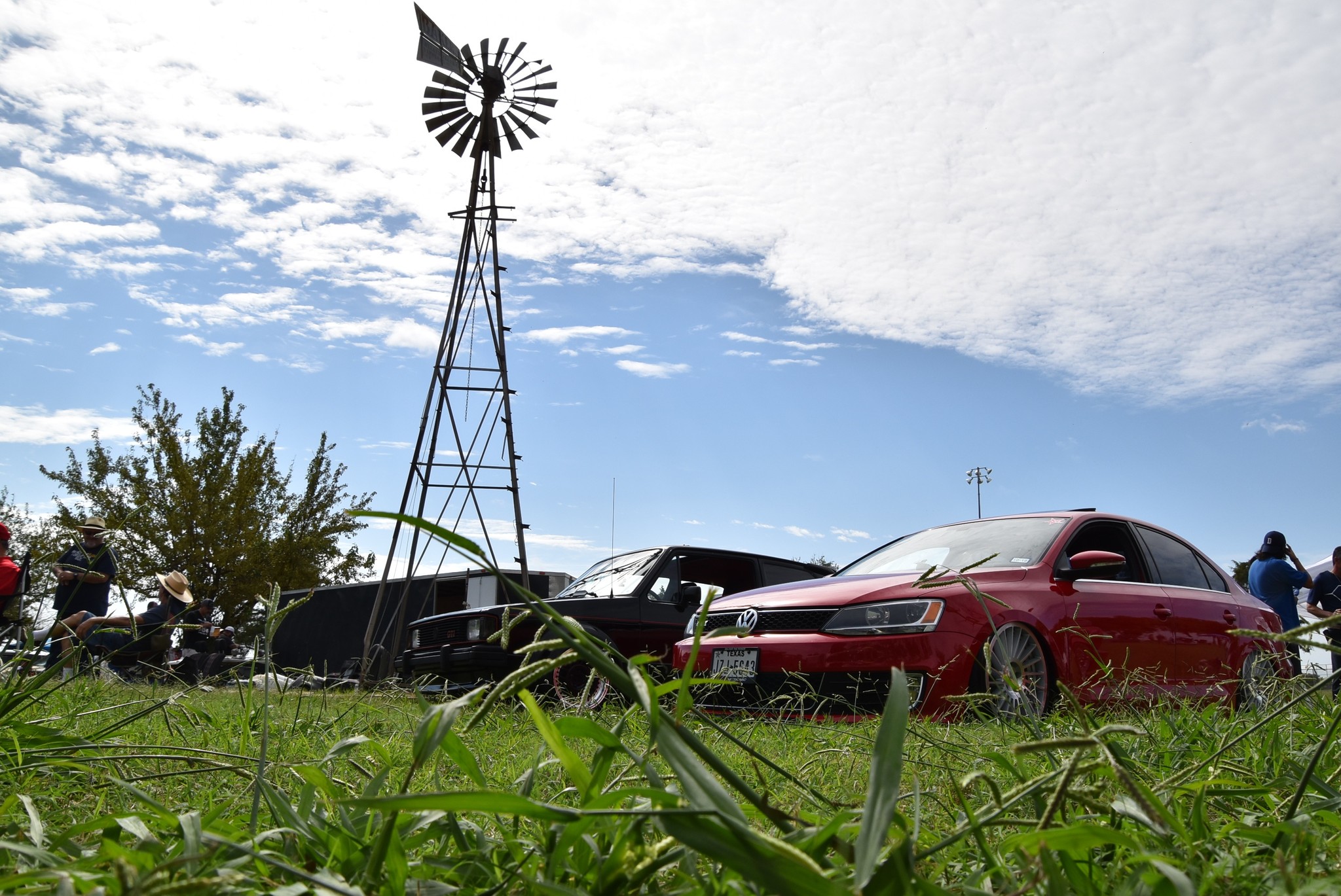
[1306,546,1341,712]
[147,601,158,611]
[43,516,119,678]
[31,570,185,683]
[219,626,239,656]
[183,598,214,653]
[0,523,21,614]
[1248,531,1313,689]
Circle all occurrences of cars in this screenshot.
[0,637,106,679]
[675,508,1298,725]
[1297,552,1341,679]
[399,545,839,713]
[161,653,271,688]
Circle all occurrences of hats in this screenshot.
[225,626,236,636]
[76,516,111,534]
[0,522,11,540]
[1261,531,1286,555]
[155,570,194,603]
[202,598,215,606]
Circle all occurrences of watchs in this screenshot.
[73,572,78,581]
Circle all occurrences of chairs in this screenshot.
[92,592,186,691]
[0,551,32,685]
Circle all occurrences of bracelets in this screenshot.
[1294,558,1298,564]
